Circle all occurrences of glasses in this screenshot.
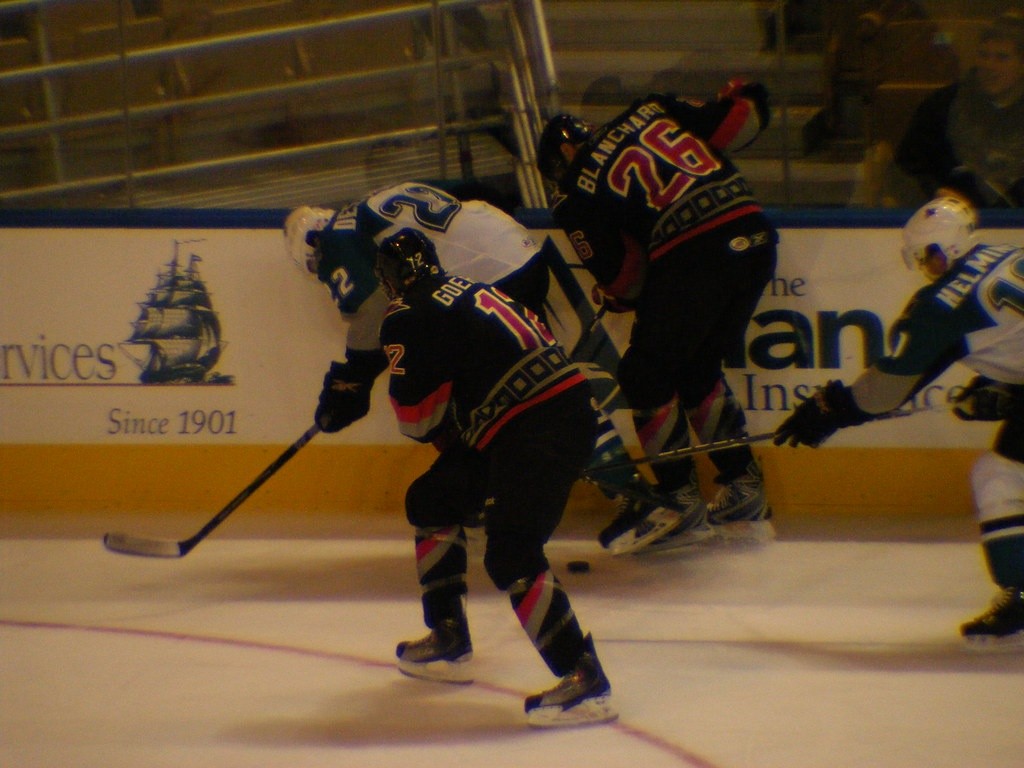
[305,254,313,270]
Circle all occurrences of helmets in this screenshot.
[282,205,336,274]
[899,196,975,282]
[375,226,446,307]
[535,112,596,195]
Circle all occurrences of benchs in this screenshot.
[506,52,959,209]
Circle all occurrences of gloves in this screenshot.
[591,283,634,313]
[772,379,876,449]
[951,374,1024,421]
[313,360,377,433]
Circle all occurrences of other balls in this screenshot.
[567,560,590,574]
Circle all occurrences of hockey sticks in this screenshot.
[476,399,964,522]
[100,357,392,561]
[569,303,608,360]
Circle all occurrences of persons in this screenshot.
[774,189,1024,646]
[884,14,1023,211]
[534,78,783,560]
[282,180,681,556]
[314,228,618,729]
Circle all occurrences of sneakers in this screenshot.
[596,432,716,557]
[957,586,1024,645]
[706,454,777,541]
[523,631,621,728]
[393,613,476,682]
